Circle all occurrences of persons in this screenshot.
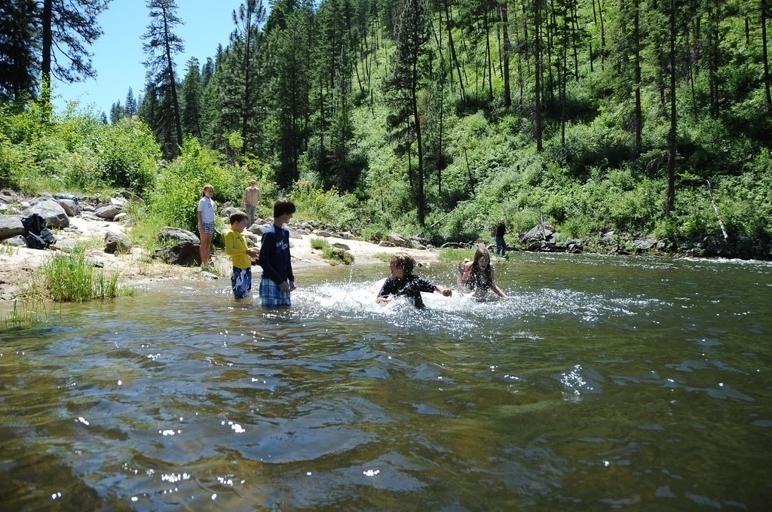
[458,246,509,301]
[224,210,258,301]
[495,222,507,256]
[197,184,216,269]
[258,199,296,309]
[244,180,260,229]
[375,252,452,313]
